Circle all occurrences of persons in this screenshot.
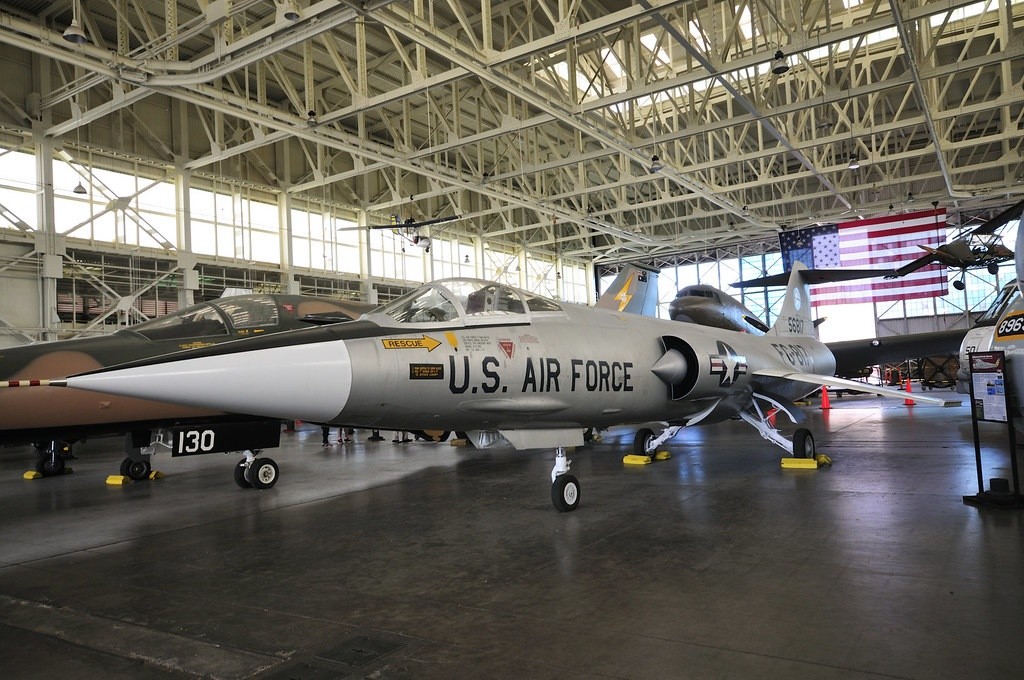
[320,426,413,449]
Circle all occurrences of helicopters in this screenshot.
[883,199,1024,290]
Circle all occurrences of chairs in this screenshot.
[465,289,494,315]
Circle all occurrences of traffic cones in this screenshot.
[819,384,834,409]
[903,378,917,405]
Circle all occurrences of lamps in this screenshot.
[63,0,87,43]
[284,0,304,21]
[307,112,318,126]
[482,172,491,185]
[651,155,662,171]
[818,117,833,130]
[888,205,896,216]
[73,128,88,194]
[907,193,915,203]
[771,0,790,74]
[742,206,750,217]
[464,254,470,264]
[556,272,562,279]
[848,154,859,169]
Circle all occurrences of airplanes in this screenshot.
[0,260,963,512]
[0,288,472,490]
[668,284,971,401]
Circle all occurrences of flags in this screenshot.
[778,208,948,308]
